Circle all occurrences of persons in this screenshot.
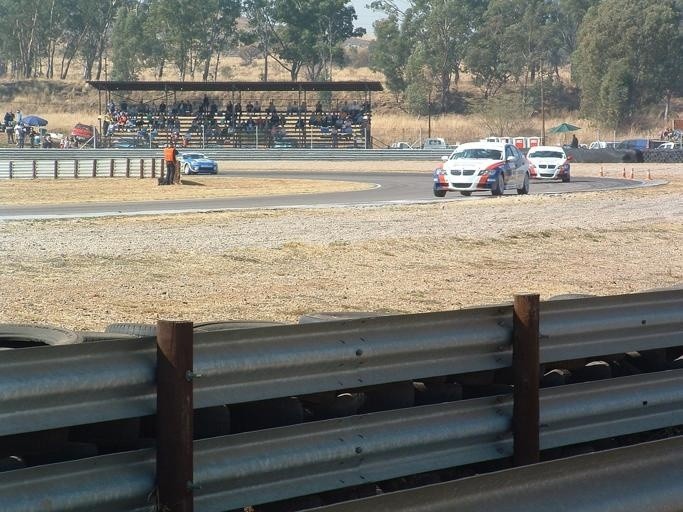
[570,134,578,149]
[42,136,52,149]
[63,136,71,149]
[164,141,179,186]
[103,92,373,149]
[3,110,40,148]
[59,139,64,148]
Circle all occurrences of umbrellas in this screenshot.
[20,116,47,127]
[548,121,582,141]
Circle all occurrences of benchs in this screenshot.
[103,111,372,148]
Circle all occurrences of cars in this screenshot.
[579,139,681,150]
[429,139,532,199]
[175,151,219,174]
[525,144,573,182]
[390,137,448,149]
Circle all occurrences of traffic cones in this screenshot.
[598,165,651,180]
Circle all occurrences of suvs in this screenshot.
[70,121,98,144]
